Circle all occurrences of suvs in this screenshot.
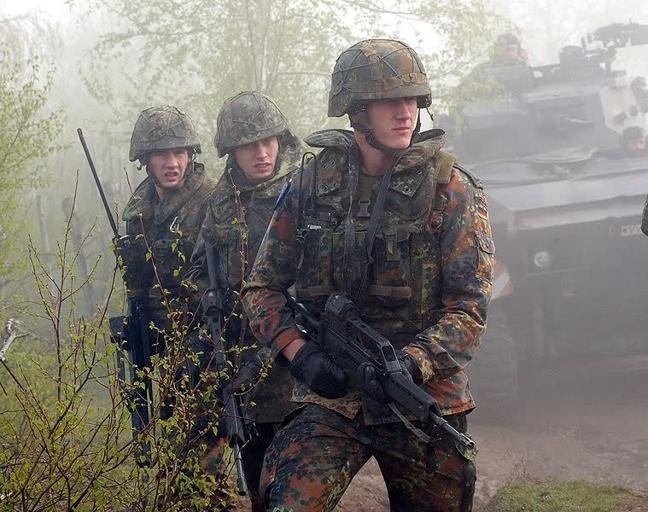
[430,44,648,413]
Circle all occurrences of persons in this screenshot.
[493,34,520,64]
[123,105,219,512]
[620,127,646,149]
[559,46,586,63]
[179,91,314,511]
[641,194,648,238]
[239,39,495,512]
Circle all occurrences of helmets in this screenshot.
[128,104,202,162]
[214,90,295,159]
[327,38,431,116]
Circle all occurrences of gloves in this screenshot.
[354,348,420,405]
[171,330,212,393]
[214,354,263,398]
[288,340,349,400]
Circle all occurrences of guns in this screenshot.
[109,239,151,468]
[281,288,476,450]
[202,241,246,496]
